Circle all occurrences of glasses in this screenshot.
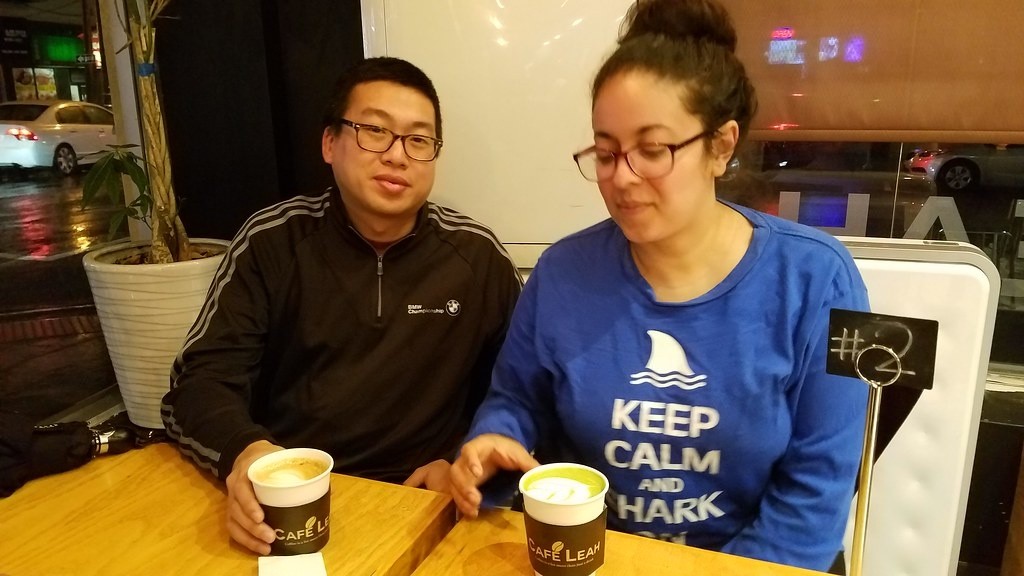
[336,116,444,162]
[575,132,708,182]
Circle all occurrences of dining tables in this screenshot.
[408,506,846,576]
[0,439,456,576]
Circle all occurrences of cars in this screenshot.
[0,97,115,174]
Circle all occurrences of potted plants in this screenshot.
[80,0,239,431]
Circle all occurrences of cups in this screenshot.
[249,447,332,557]
[516,460,611,576]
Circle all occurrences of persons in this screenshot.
[449,0,870,574]
[160,58,525,561]
